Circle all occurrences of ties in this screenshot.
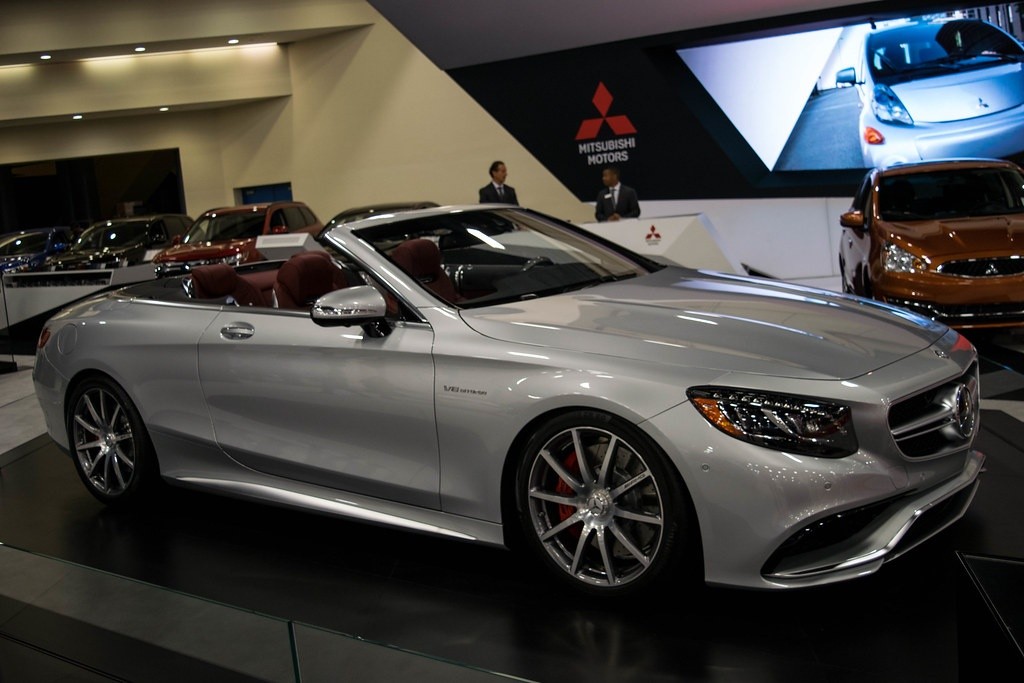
[611,188,616,210]
[499,187,502,196]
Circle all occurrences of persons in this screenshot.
[595,166,640,223]
[479,161,518,206]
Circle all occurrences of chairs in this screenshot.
[272,254,336,307]
[882,177,914,215]
[391,238,457,304]
[943,174,985,214]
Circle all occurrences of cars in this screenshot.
[838,159,1024,338]
[0,228,87,275]
[836,16,1024,170]
[310,201,441,248]
[154,203,326,278]
[42,213,211,273]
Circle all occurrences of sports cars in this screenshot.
[34,206,986,614]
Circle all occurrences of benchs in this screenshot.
[189,251,344,308]
